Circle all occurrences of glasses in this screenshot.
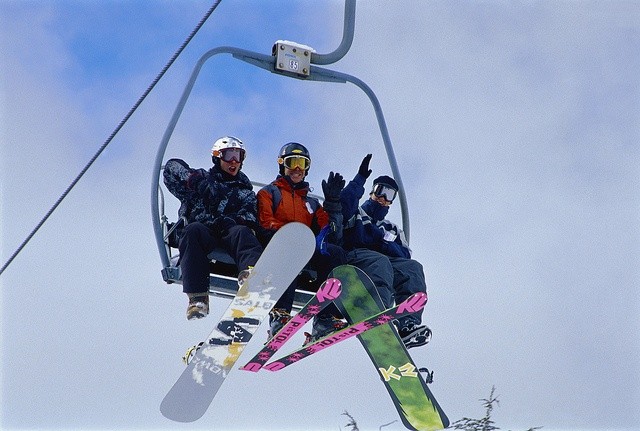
[282,154,311,170]
[373,182,397,201]
[219,146,247,163]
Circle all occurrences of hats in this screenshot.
[370,175,399,203]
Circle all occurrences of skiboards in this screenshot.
[238,279,428,372]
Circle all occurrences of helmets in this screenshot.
[277,141,311,177]
[211,134,247,161]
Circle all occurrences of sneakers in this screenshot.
[238,268,250,286]
[267,313,293,338]
[399,323,433,348]
[310,315,349,341]
[187,295,209,320]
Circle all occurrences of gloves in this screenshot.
[187,172,217,201]
[321,170,345,212]
[359,152,372,177]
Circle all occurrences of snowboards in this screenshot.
[160,222,316,422]
[327,265,449,430]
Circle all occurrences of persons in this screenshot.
[163,136,264,320]
[257,143,347,341]
[342,153,432,349]
